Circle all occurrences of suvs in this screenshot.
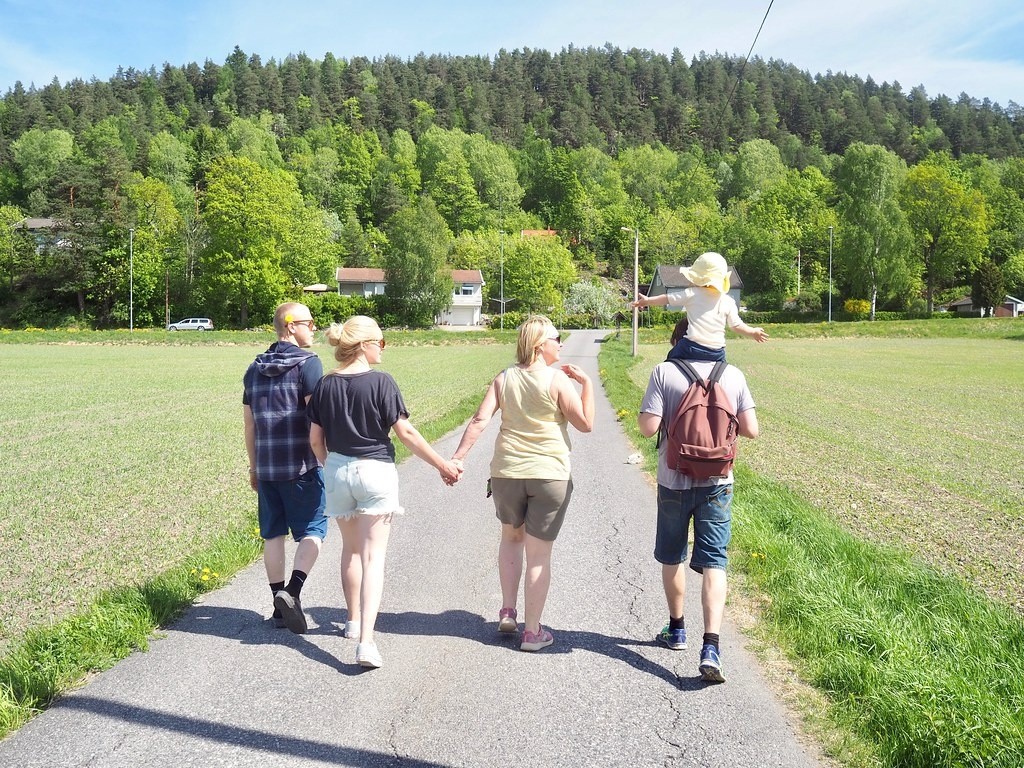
[165,318,214,331]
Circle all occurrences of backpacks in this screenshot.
[664,358,740,480]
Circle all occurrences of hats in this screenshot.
[679,252,732,294]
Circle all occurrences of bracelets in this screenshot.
[247,465,257,473]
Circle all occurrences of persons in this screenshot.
[637,319,758,681]
[631,251,769,364]
[306,315,464,667]
[242,302,329,633]
[443,315,595,653]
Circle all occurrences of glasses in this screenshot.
[285,318,313,332]
[546,335,561,344]
[352,338,385,350]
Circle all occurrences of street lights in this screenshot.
[621,226,639,357]
[827,225,834,322]
[129,228,134,332]
[499,231,505,331]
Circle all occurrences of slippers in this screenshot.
[270,616,286,628]
[274,591,307,633]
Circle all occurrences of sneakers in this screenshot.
[497,608,520,633]
[355,641,382,667]
[659,623,687,649]
[346,619,361,639]
[699,644,727,682]
[520,624,553,650]
